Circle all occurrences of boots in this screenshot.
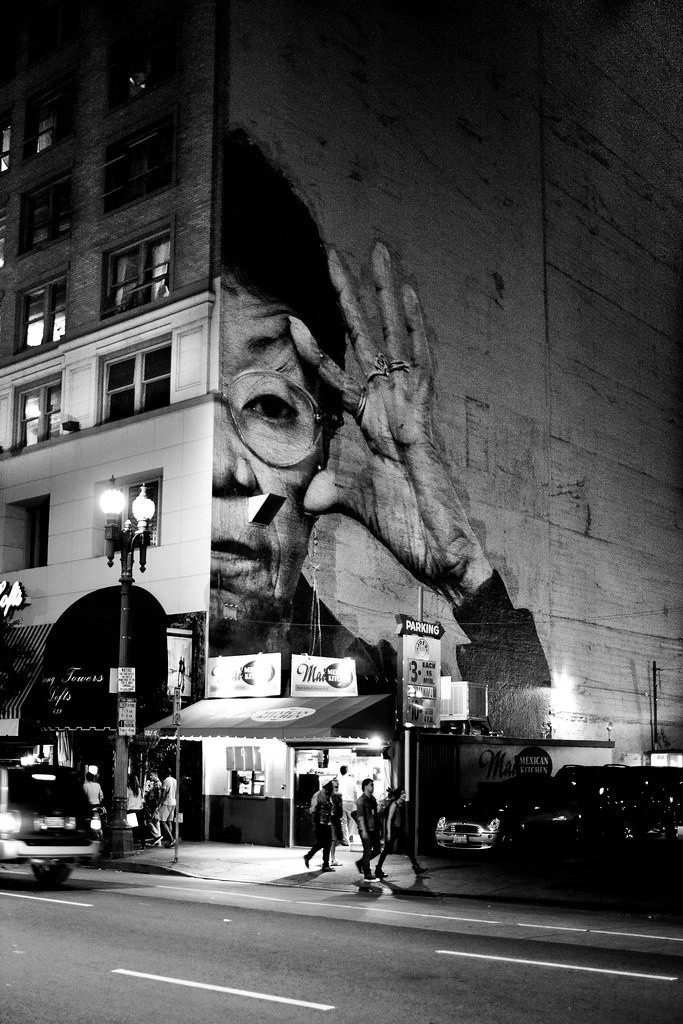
[375,865,388,877]
[412,865,428,876]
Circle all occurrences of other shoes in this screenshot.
[164,839,176,848]
[138,846,145,851]
[356,861,363,873]
[363,874,380,882]
[322,866,336,872]
[331,861,342,866]
[304,855,309,869]
[152,836,163,846]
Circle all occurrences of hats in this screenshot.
[322,780,333,789]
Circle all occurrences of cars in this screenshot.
[535,764,683,840]
[1,764,101,886]
[433,775,556,855]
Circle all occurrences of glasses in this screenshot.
[333,786,339,788]
[221,369,338,469]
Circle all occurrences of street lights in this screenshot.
[100,475,155,861]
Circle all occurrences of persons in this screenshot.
[82,767,177,846]
[304,765,429,882]
